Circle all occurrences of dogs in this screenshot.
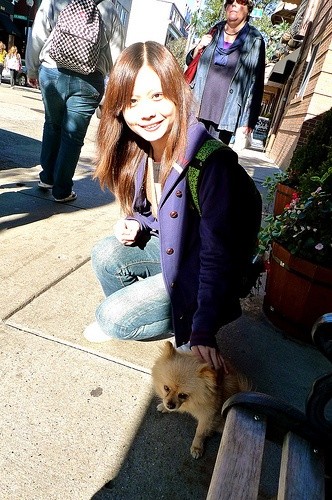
[151,341,253,459]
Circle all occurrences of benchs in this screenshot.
[206,313,332,500]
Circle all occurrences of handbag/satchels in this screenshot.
[185,27,218,84]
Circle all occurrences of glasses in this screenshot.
[226,0,249,5]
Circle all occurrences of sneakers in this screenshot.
[37,180,53,188]
[54,191,78,202]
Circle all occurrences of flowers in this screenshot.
[252,166,332,276]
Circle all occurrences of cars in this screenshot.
[2,59,28,87]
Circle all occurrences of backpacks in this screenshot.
[187,140,265,299]
[50,1,104,75]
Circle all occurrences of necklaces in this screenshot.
[224,24,244,36]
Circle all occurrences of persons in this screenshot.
[83,40,263,375]
[25,0,126,203]
[0,41,7,84]
[4,46,22,87]
[183,0,265,146]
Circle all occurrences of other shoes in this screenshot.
[82,320,111,343]
[174,342,191,353]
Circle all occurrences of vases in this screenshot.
[273,182,302,223]
[263,240,332,336]
